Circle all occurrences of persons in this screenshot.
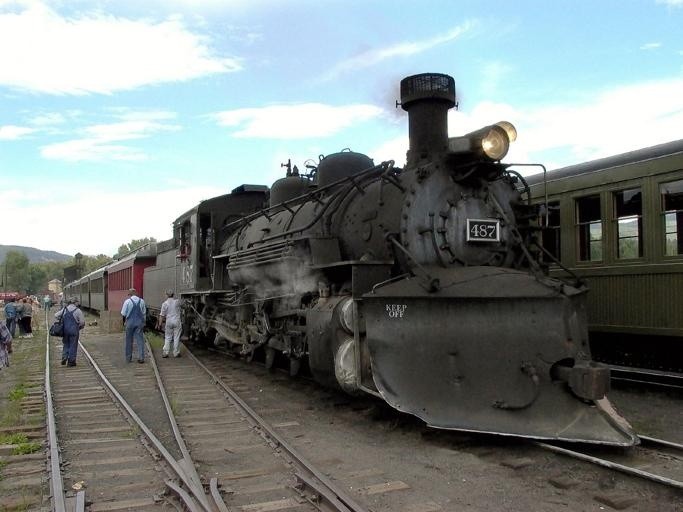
[120,289,148,363]
[4,297,39,339]
[44,294,52,311]
[0,320,14,370]
[155,289,184,359]
[54,296,85,366]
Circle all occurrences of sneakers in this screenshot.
[68,363,76,366]
[139,360,143,362]
[162,354,168,357]
[24,333,33,338]
[61,358,67,365]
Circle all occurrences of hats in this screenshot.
[166,290,174,294]
[69,298,79,303]
[128,289,135,295]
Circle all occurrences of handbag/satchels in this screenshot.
[49,323,64,337]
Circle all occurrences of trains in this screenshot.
[508,140,683,368]
[48,73,640,449]
